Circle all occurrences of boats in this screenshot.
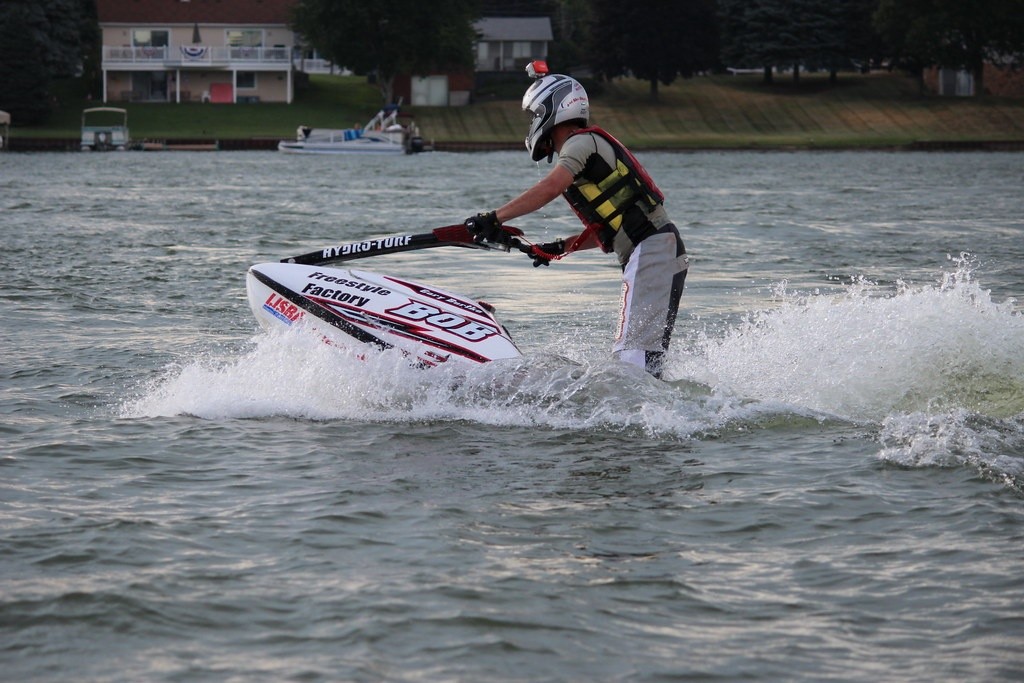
[246,222,530,402]
[80,107,131,151]
[278,97,434,154]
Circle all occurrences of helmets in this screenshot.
[522,60,589,163]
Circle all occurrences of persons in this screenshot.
[466,73,688,380]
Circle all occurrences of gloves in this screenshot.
[464,209,501,235]
[528,238,565,267]
